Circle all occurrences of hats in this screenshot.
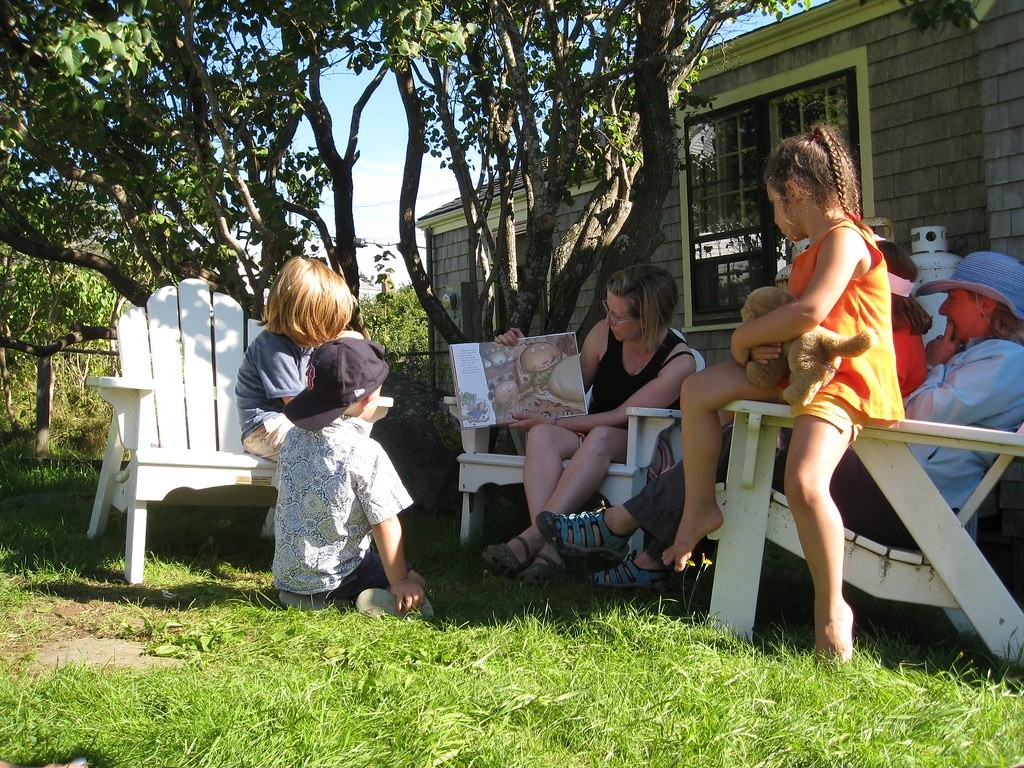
[873,234,915,297]
[283,337,390,431]
[915,251,1024,321]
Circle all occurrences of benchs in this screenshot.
[705,387,1024,682]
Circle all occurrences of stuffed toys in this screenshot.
[741,287,878,406]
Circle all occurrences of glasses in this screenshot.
[602,299,634,324]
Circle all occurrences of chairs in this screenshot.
[442,326,706,566]
[80,275,395,590]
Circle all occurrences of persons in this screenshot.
[481,265,698,584]
[536,240,932,586]
[271,337,435,616]
[830,251,1024,550]
[235,256,376,539]
[662,123,908,668]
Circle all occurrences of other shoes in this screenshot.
[355,588,434,618]
[279,590,327,610]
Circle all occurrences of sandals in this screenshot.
[535,499,634,562]
[591,550,668,591]
[518,553,568,590]
[481,536,533,573]
